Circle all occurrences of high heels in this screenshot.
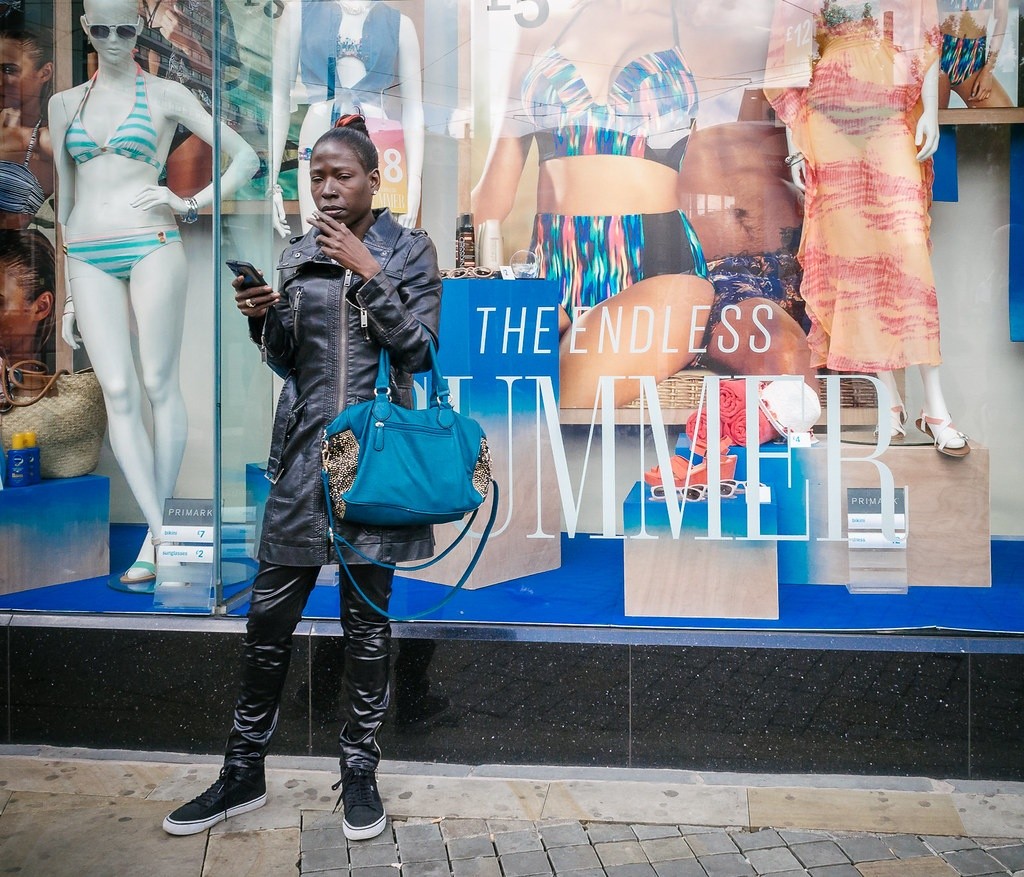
[120,528,156,582]
[643,437,738,491]
[151,535,191,592]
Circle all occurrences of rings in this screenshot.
[246,298,256,308]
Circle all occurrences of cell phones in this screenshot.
[225,259,273,297]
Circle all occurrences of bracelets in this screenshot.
[265,185,283,199]
[785,152,805,167]
[180,198,199,224]
[62,295,75,315]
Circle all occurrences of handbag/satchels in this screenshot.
[0,360,107,479]
[322,325,491,526]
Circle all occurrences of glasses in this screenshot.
[649,480,766,502]
[84,14,140,39]
[439,265,495,280]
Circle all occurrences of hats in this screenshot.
[760,372,821,445]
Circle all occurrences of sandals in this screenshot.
[916,409,970,458]
[873,401,908,439]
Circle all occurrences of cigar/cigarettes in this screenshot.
[312,213,322,222]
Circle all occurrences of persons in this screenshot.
[133,0,246,198]
[266,0,425,238]
[2,0,57,376]
[937,0,1014,108]
[164,113,443,840]
[763,0,971,457]
[47,0,260,610]
[470,1,820,408]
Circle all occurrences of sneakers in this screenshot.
[331,767,387,841]
[162,770,267,835]
